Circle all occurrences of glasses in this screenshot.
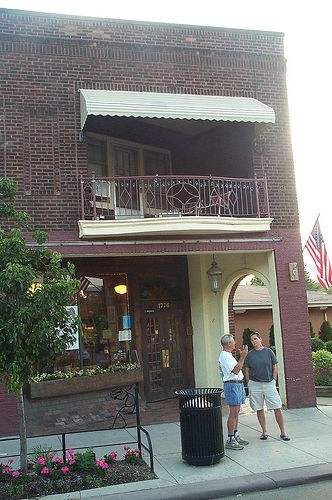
[250,332,260,336]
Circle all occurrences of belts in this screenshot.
[224,380,244,384]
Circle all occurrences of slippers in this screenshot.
[260,433,268,440]
[280,433,290,440]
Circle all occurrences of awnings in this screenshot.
[79,87,277,137]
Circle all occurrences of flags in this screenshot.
[306,219,332,291]
[69,277,92,305]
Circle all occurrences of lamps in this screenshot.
[206,254,223,295]
[114,281,127,294]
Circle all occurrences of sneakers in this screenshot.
[225,435,249,450]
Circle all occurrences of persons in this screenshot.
[219,334,249,451]
[244,332,291,441]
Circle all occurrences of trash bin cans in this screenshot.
[176,388,226,466]
